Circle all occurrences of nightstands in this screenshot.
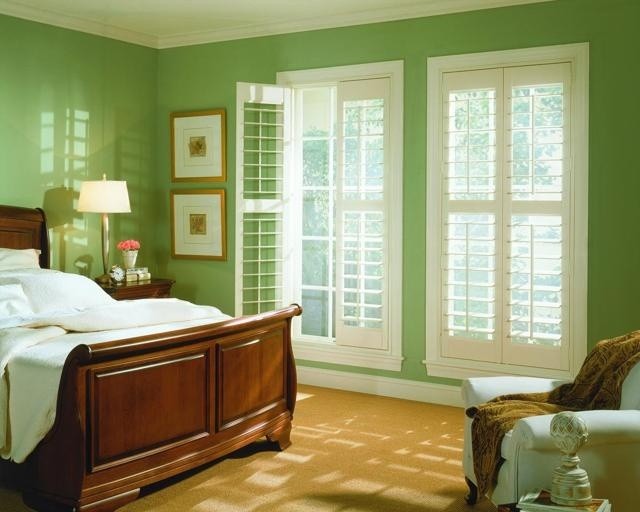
[95,277,176,301]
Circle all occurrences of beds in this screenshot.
[0,205,303,512]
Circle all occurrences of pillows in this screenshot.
[0,247,42,272]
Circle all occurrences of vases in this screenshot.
[122,250,138,270]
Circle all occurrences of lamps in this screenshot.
[77,173,132,283]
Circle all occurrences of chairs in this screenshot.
[462,330,640,512]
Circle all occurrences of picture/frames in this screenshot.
[170,109,227,182]
[170,189,227,261]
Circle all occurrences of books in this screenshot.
[515,485,612,512]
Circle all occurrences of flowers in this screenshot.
[117,239,140,251]
